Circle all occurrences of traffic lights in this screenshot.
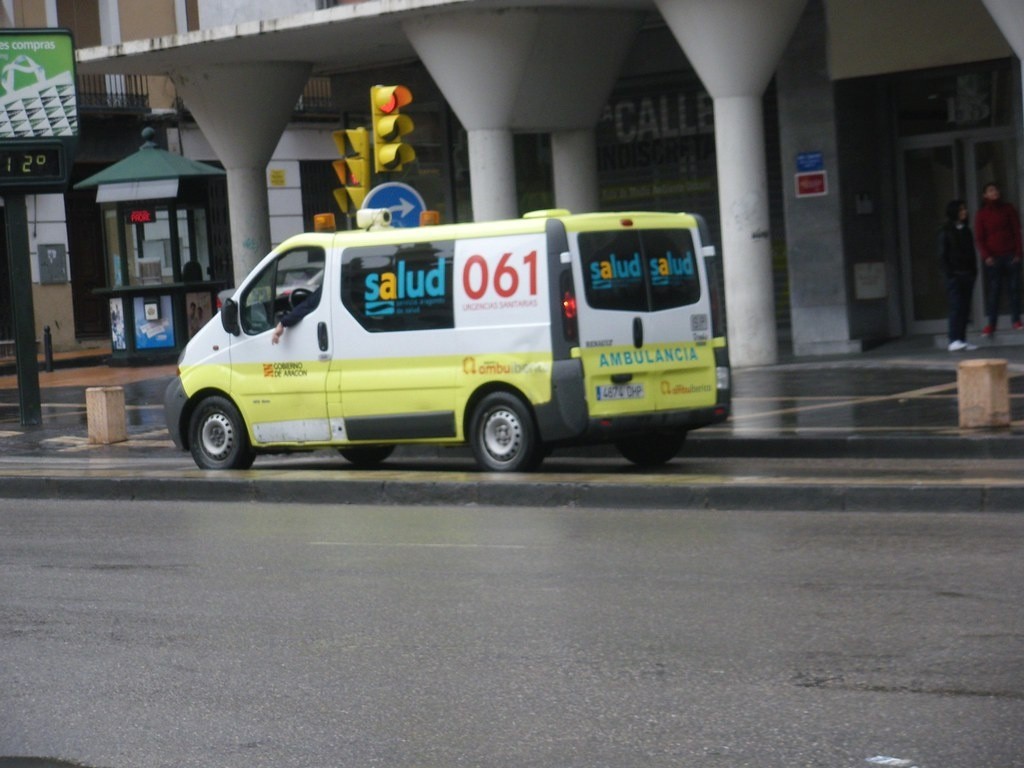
[370,84,417,176]
[332,127,372,214]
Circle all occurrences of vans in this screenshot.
[158,208,732,477]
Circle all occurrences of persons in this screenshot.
[272,287,322,343]
[187,303,206,339]
[938,200,980,352]
[974,183,1024,337]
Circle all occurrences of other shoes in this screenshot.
[947,340,966,352]
[1012,322,1023,329]
[961,342,978,351]
[981,325,993,336]
